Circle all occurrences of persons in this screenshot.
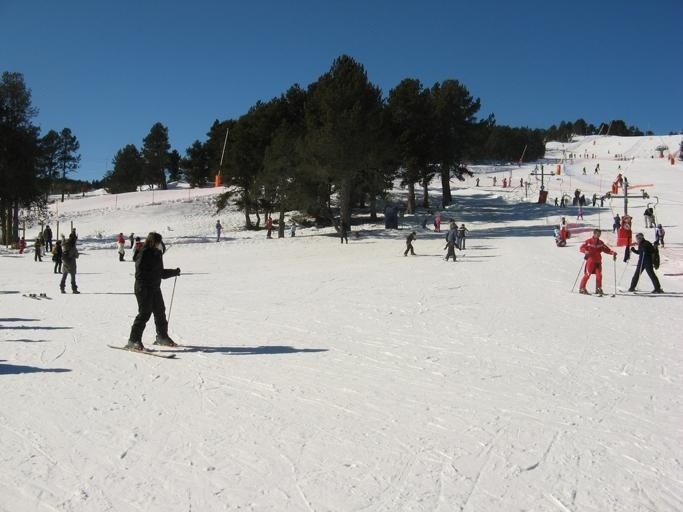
[403,231,416,255]
[128,232,135,248]
[627,233,664,294]
[582,167,587,175]
[644,208,653,228]
[33,225,79,294]
[124,231,180,350]
[593,167,598,174]
[290,221,296,237]
[115,233,126,261]
[655,224,665,246]
[553,197,558,207]
[559,216,570,244]
[263,214,272,239]
[578,228,617,294]
[215,219,223,242]
[17,236,26,253]
[432,211,469,261]
[131,237,143,261]
[573,189,610,220]
[596,163,600,170]
[611,213,620,232]
[559,197,565,208]
[475,177,480,186]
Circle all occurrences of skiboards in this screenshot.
[618,290,683,297]
[110,342,211,358]
[582,293,615,297]
[25,293,49,300]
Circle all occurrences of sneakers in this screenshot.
[579,287,588,294]
[128,340,144,350]
[156,335,177,346]
[596,288,603,294]
